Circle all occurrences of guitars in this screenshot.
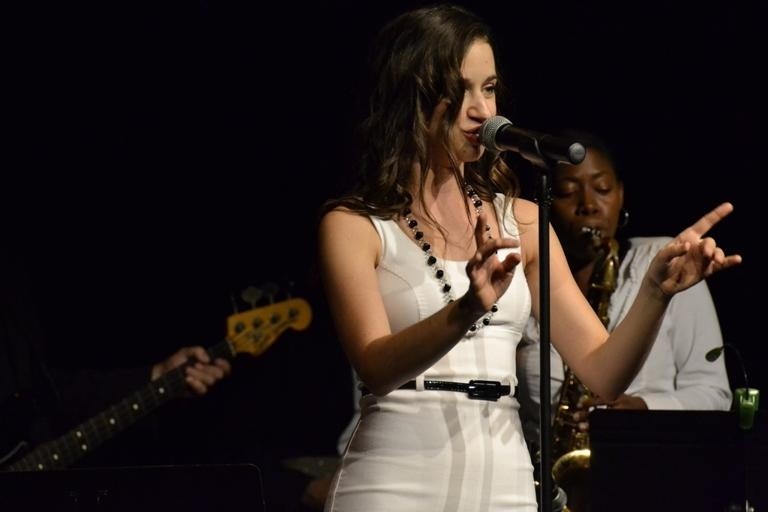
[0,280,314,472]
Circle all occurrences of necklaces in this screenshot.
[397,182,503,335]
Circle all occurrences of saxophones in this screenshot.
[533,226,620,512]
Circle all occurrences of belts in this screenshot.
[357,379,520,402]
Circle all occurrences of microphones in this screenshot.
[479,115,586,166]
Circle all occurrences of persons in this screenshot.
[1,298,233,511]
[316,2,744,511]
[515,121,734,411]
